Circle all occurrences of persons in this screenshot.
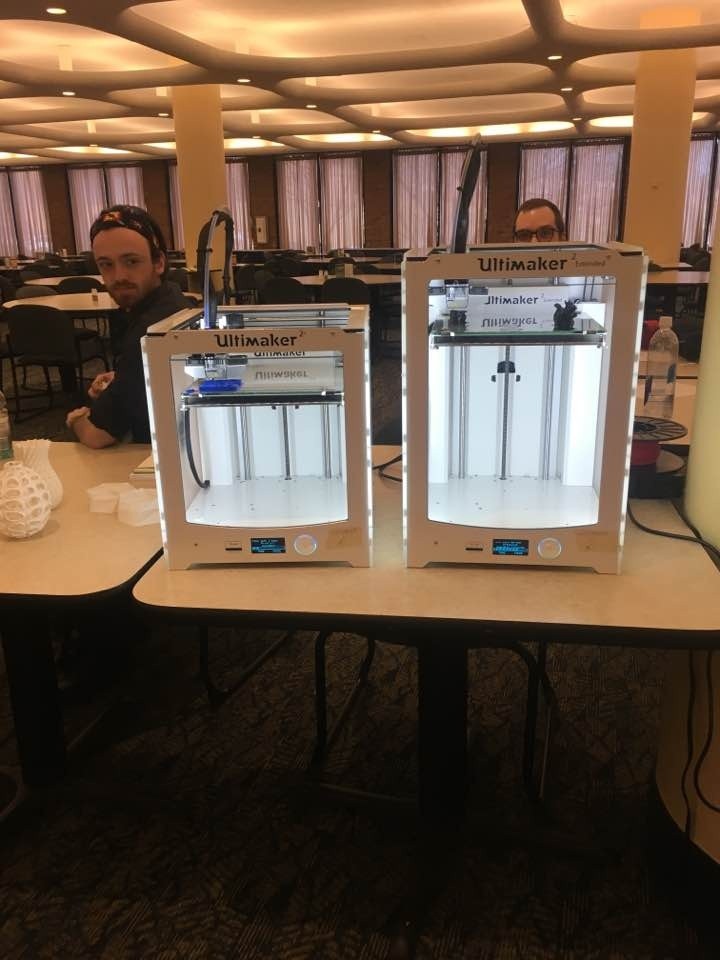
[511,198,565,244]
[65,204,199,450]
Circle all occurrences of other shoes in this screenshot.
[53,635,130,693]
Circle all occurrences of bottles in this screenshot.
[643,316,679,420]
[0,391,14,471]
[91,288,98,305]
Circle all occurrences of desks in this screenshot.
[290,270,404,287]
[0,423,160,790]
[25,273,154,288]
[641,269,714,316]
[166,263,221,274]
[648,260,697,269]
[345,245,446,251]
[302,255,384,264]
[131,439,720,837]
[2,290,202,401]
[0,262,28,273]
[14,254,84,265]
[231,247,296,255]
[229,261,266,269]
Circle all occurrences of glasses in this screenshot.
[513,228,560,242]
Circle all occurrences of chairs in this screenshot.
[0,240,720,415]
